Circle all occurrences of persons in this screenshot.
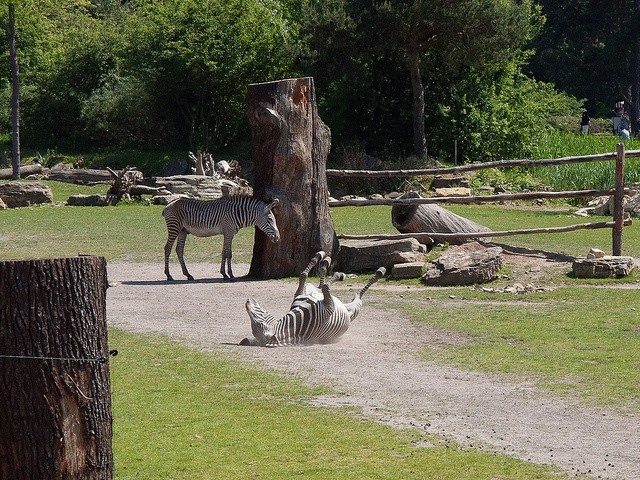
[610,108,624,136]
[581,111,592,135]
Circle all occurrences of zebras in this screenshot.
[238,250,388,347]
[161,193,281,281]
